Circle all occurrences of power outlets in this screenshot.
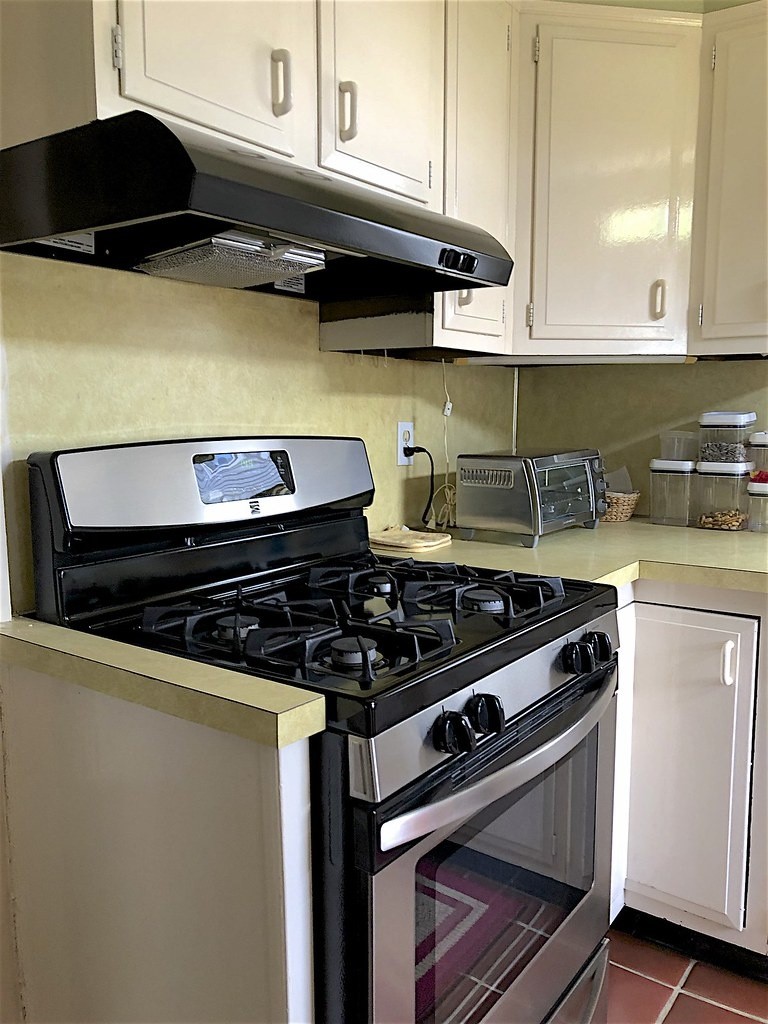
[397,421,413,466]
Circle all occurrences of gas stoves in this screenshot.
[27,436,621,803]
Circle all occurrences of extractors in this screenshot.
[0,110,516,304]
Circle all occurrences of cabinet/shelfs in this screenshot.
[317,1,520,361]
[524,1,685,363]
[628,602,760,935]
[93,0,445,213]
[688,11,768,360]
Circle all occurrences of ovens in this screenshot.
[455,445,610,549]
[310,648,617,1024]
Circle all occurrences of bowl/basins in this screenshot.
[648,409,768,533]
